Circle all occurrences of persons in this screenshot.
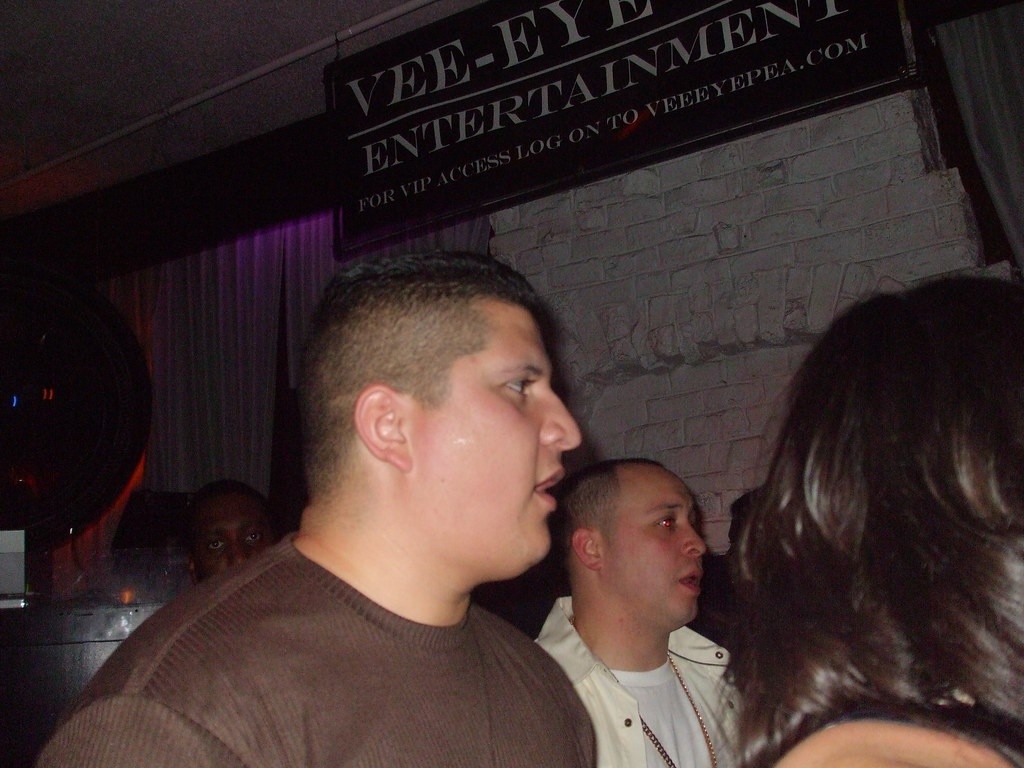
[36,251,598,767]
[533,457,774,748]
[706,269,1024,767]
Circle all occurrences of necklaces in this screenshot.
[601,652,718,766]
